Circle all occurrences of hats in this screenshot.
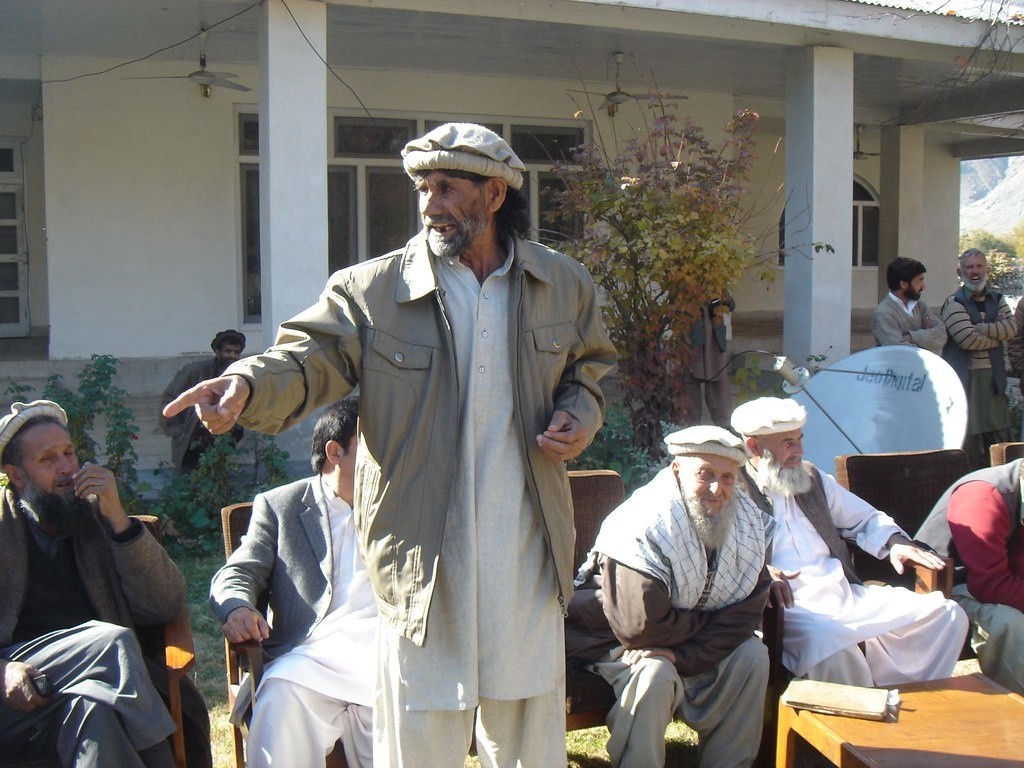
[664,425,750,467]
[730,396,807,434]
[0,399,68,473]
[212,329,246,353]
[400,122,525,191]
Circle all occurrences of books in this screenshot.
[781,677,889,721]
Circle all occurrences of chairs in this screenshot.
[989,442,1024,467]
[126,515,195,768]
[567,470,626,733]
[836,448,975,598]
[221,502,254,768]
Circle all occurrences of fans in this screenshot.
[120,30,253,92]
[565,52,689,111]
[853,125,882,162]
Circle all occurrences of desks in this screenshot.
[776,673,1024,768]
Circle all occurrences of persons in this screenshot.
[564,424,772,768]
[0,400,187,768]
[669,289,736,431]
[940,248,1017,469]
[207,396,377,768]
[869,256,948,357]
[164,123,618,768]
[914,458,1024,696]
[1007,296,1024,441]
[159,329,246,475]
[730,397,969,688]
